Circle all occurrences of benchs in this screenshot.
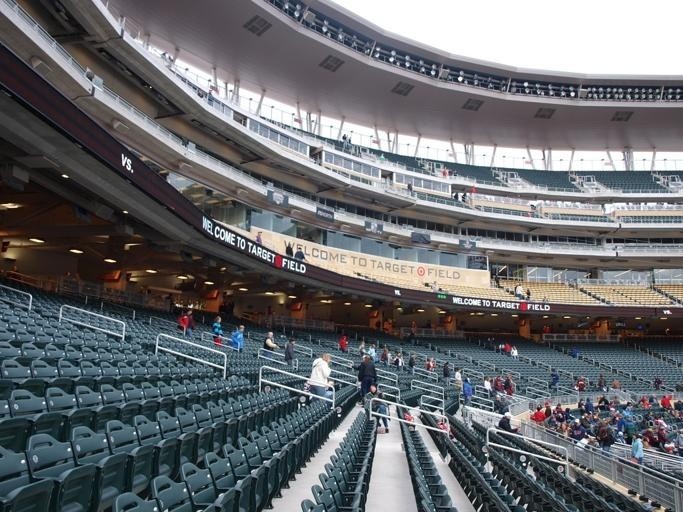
[0,279,683,512]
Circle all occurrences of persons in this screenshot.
[8,265,19,279]
[63,270,72,287]
[255,229,262,244]
[342,133,347,148]
[0,268,8,279]
[530,204,534,218]
[448,169,452,178]
[161,49,168,60]
[493,273,501,287]
[286,242,292,256]
[134,281,683,450]
[454,192,458,201]
[533,206,538,218]
[453,168,457,176]
[407,180,413,195]
[630,433,644,465]
[166,55,174,68]
[461,194,465,203]
[205,88,215,102]
[294,248,308,263]
[443,169,447,178]
